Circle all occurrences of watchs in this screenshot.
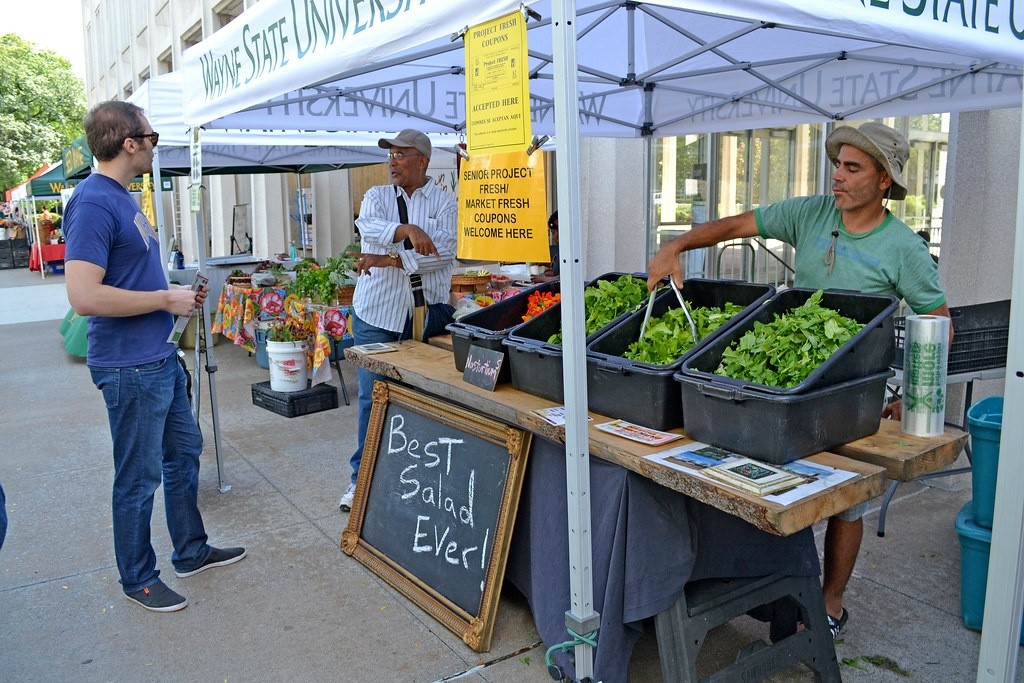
[389,251,398,267]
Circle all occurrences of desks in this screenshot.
[162,262,259,315]
[28,244,65,276]
[342,337,968,683]
[208,282,521,405]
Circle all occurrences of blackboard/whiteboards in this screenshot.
[340,381,533,652]
[462,345,505,391]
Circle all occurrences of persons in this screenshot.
[0,202,8,219]
[339,129,458,512]
[42,206,53,237]
[499,210,560,284]
[62,101,247,612]
[646,122,954,641]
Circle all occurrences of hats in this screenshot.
[378,129,432,161]
[824,122,910,201]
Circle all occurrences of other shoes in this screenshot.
[338,482,356,510]
[123,578,187,612]
[827,606,850,640]
[174,546,246,579]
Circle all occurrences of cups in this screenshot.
[530,266,539,283]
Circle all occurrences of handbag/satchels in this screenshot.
[398,303,458,342]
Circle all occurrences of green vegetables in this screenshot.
[710,287,867,390]
[618,299,751,364]
[545,272,647,347]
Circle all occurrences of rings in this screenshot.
[359,258,365,263]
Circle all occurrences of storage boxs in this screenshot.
[10,228,25,239]
[889,299,1011,375]
[954,395,1024,648]
[252,327,275,368]
[442,271,900,463]
[175,310,219,349]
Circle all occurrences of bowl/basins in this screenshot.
[491,279,514,289]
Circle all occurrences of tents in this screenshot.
[5,135,179,280]
[92,69,556,359]
[182,0,1024,683]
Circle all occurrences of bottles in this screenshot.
[290,241,296,260]
[175,250,185,269]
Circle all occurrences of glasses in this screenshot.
[385,152,424,160]
[122,132,159,147]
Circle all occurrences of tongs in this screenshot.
[637,274,700,345]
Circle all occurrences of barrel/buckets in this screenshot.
[266,338,309,392]
